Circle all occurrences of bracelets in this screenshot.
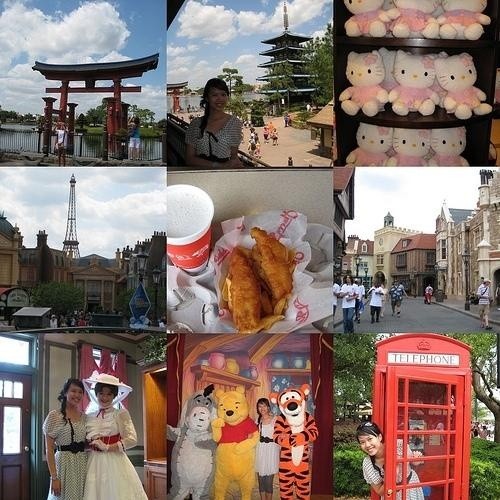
[51,476,57,481]
[213,161,218,168]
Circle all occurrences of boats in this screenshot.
[31,127,40,132]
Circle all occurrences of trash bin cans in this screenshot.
[433,289,447,302]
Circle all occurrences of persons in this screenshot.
[355,421,425,500]
[256,398,276,500]
[127,115,140,160]
[177,105,203,122]
[334,275,412,333]
[338,1,492,166]
[425,285,433,305]
[495,285,500,309]
[187,78,244,168]
[52,122,69,165]
[245,112,291,154]
[82,369,147,500]
[477,278,493,330]
[54,308,120,331]
[471,421,488,438]
[41,378,88,500]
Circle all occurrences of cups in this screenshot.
[167,183,216,278]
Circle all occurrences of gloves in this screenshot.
[88,439,109,452]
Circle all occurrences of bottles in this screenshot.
[209,352,308,379]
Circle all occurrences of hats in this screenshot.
[84,373,133,393]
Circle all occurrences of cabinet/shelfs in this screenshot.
[333,0,500,166]
[141,362,167,500]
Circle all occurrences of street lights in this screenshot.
[150,263,163,326]
[460,248,472,310]
[355,253,363,277]
[363,264,369,299]
[133,244,151,284]
